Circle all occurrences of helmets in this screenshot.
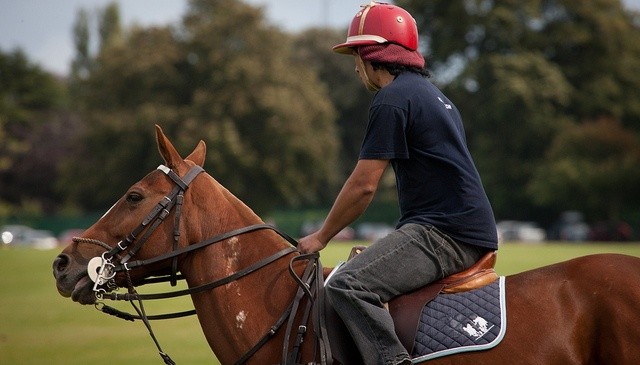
[333,2,420,54]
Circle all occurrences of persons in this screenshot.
[298,2,498,364]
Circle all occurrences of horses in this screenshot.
[51,126,640,365]
